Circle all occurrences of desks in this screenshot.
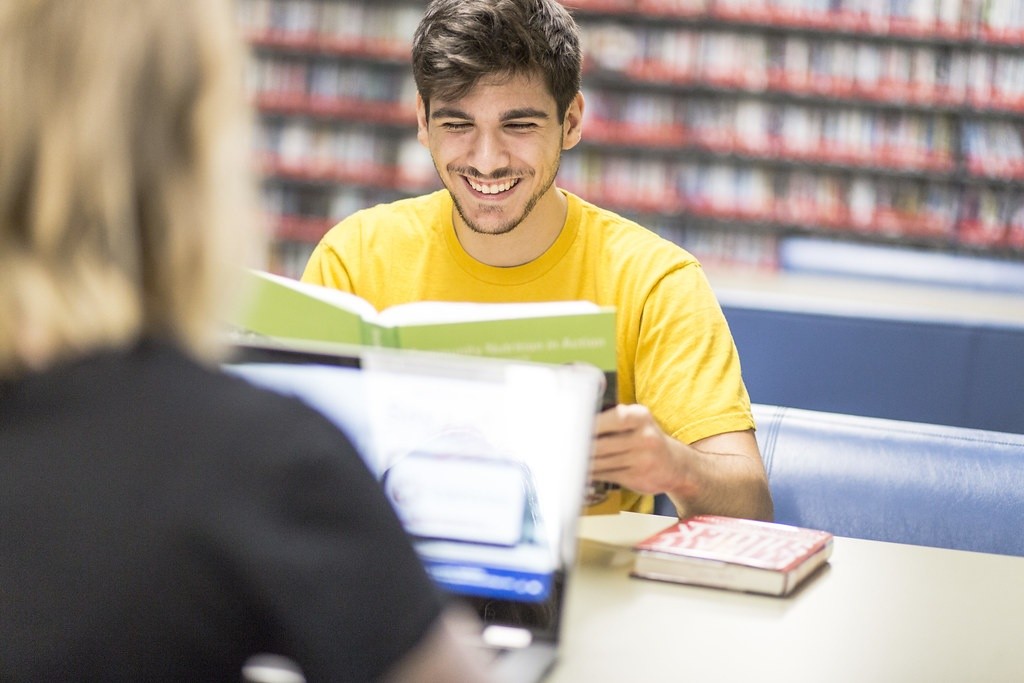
[243,507,1024,682]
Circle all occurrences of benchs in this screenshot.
[656,401,1023,558]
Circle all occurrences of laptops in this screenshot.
[214,329,607,683]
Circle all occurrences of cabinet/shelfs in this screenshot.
[236,1,1023,285]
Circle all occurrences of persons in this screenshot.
[0,0,490,683]
[298,1,773,523]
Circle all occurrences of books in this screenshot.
[238,268,624,520]
[630,514,834,597]
